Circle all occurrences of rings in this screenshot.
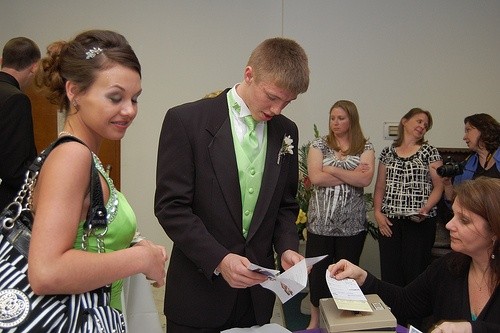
[435,325,443,332]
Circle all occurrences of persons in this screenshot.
[305,100,375,333]
[0,29,168,333]
[374,107,444,288]
[330,176,500,333]
[0,37,42,214]
[155,37,310,333]
[434,113,500,212]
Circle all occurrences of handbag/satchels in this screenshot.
[0,135,127,333]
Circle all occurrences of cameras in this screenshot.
[436,157,463,178]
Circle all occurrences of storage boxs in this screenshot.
[319,294,398,333]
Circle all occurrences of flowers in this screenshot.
[277,134,295,165]
[294,125,381,237]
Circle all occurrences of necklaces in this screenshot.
[473,263,488,292]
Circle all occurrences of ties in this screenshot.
[239,115,261,163]
